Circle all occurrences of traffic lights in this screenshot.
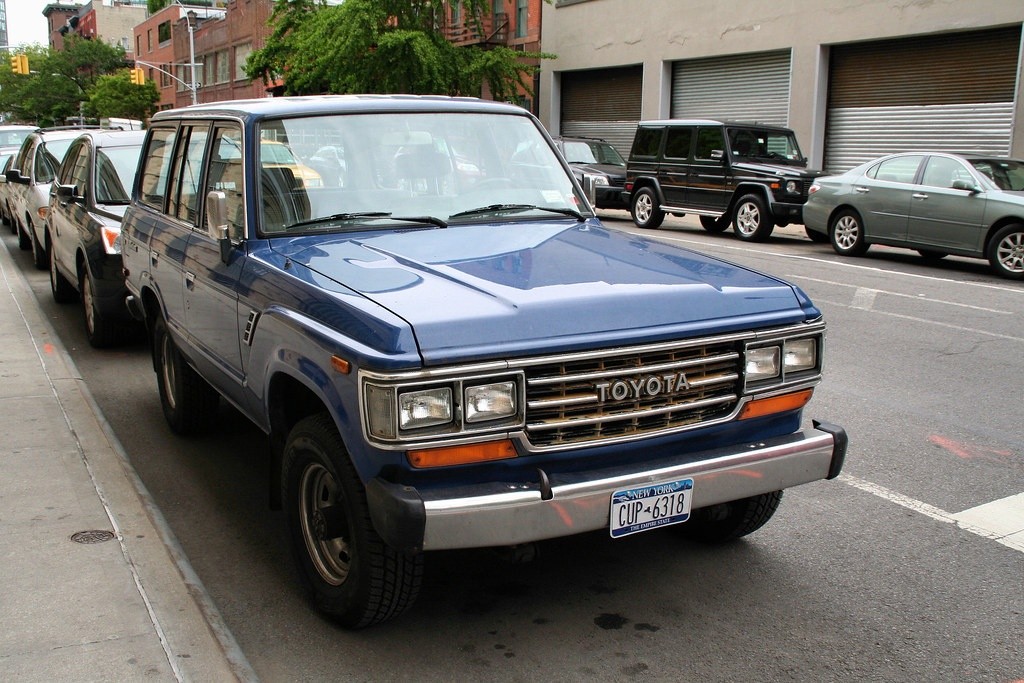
[130,69,139,84]
[10,54,22,74]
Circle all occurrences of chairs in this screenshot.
[390,143,464,217]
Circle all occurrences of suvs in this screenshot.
[620,117,832,243]
[117,90,850,632]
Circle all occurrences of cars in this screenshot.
[309,146,346,190]
[42,126,240,350]
[801,150,1024,280]
[1,125,126,270]
[508,134,628,210]
[233,140,325,189]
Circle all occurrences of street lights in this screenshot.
[58,15,85,129]
